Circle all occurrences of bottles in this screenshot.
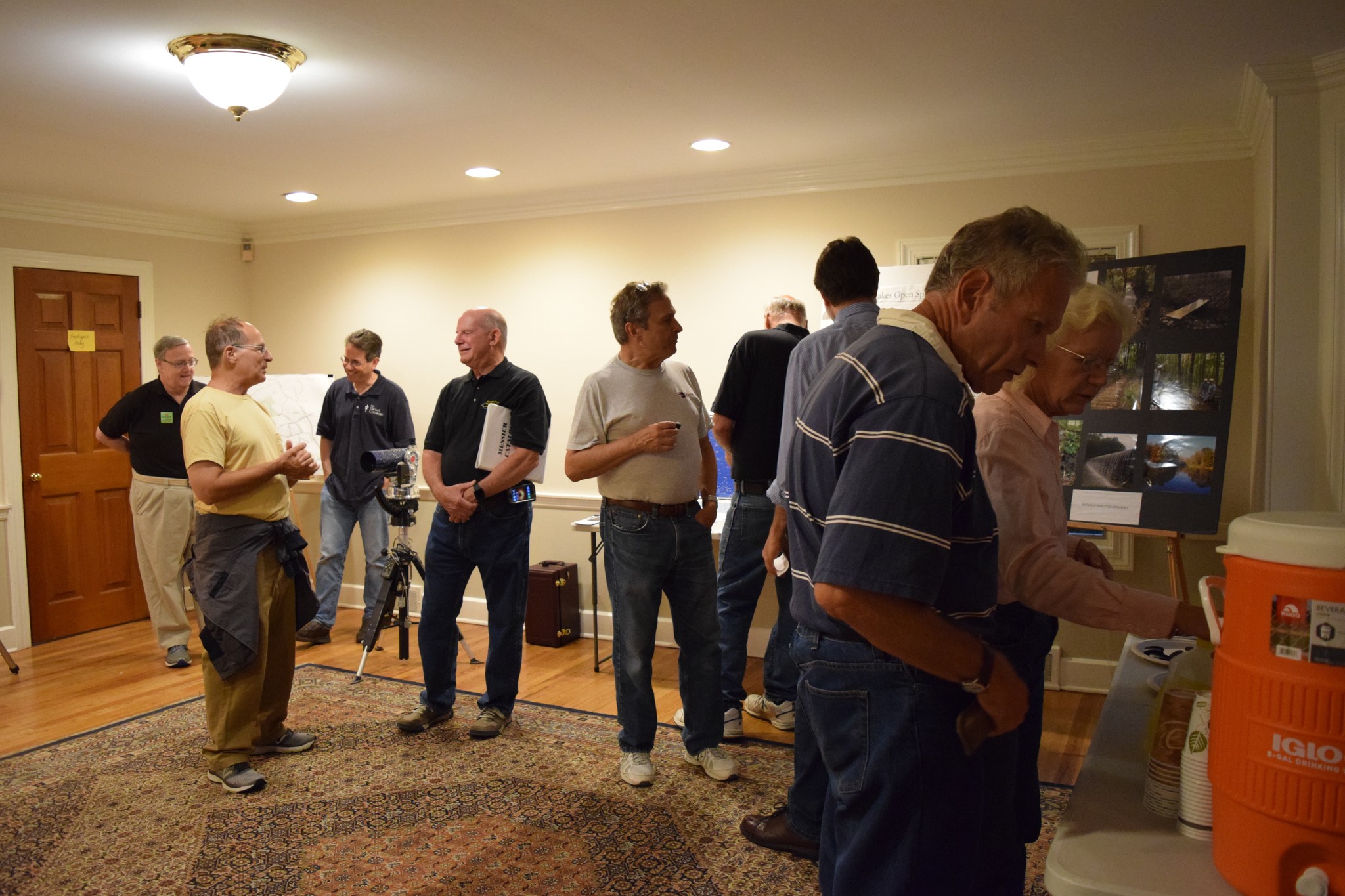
[1143,639,1215,762]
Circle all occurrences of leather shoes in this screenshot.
[739,801,820,861]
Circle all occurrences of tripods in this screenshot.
[351,495,483,685]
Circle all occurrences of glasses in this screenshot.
[1056,345,1124,376]
[163,357,198,369]
[232,343,268,355]
[631,282,650,296]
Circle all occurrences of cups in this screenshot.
[1143,687,1196,818]
[1176,689,1213,840]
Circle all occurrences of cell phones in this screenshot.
[507,483,536,505]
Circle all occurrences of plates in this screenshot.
[1130,635,1198,663]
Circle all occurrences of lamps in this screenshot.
[169,34,306,120]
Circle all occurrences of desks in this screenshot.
[1042,630,1244,894]
[569,502,732,673]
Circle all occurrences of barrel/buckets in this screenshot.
[1198,510,1344,896]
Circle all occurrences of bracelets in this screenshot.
[702,495,718,509]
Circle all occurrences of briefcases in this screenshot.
[525,559,581,648]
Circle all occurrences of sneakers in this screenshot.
[619,750,654,786]
[745,694,795,730]
[166,645,192,668]
[207,762,267,793]
[469,706,512,737]
[684,746,739,781]
[250,728,315,755]
[674,707,744,738]
[396,704,454,731]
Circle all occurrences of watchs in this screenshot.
[958,640,995,693]
[473,482,486,502]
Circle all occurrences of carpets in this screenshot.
[0,659,1077,896]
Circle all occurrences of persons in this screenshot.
[396,306,552,739]
[295,328,417,643]
[180,316,321,793]
[96,335,208,667]
[673,295,813,737]
[784,205,1091,896]
[565,280,739,786]
[973,283,1210,896]
[749,236,910,730]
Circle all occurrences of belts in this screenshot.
[131,469,191,488]
[605,496,694,516]
[733,480,768,495]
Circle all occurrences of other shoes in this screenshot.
[294,621,332,644]
[355,616,381,641]
